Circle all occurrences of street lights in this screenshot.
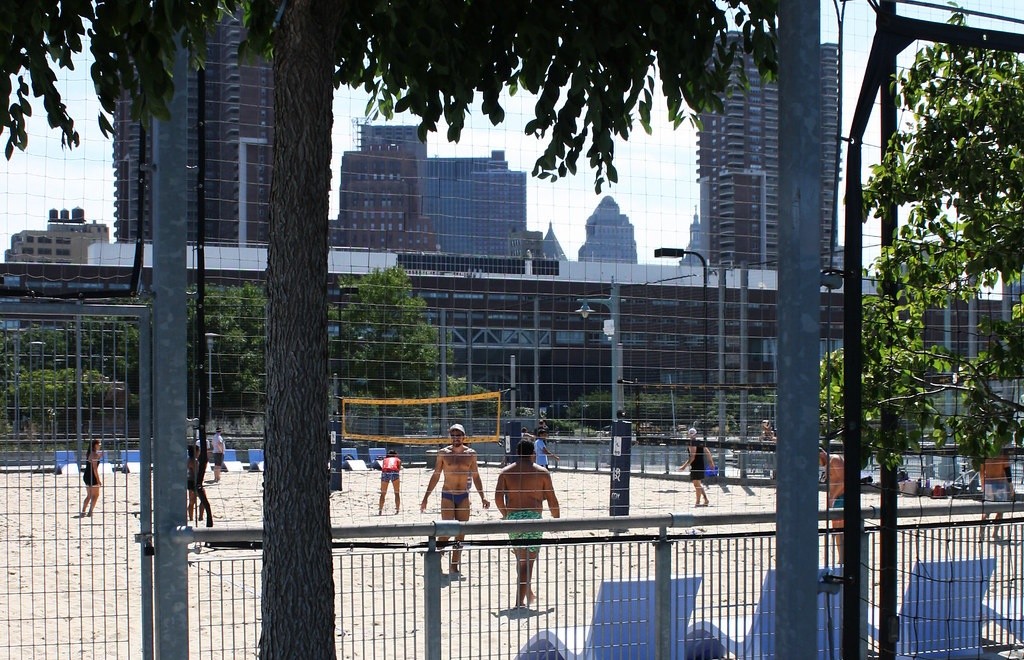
[330,285,359,492]
[573,276,633,532]
[653,247,708,448]
[204,332,220,435]
[717,166,768,270]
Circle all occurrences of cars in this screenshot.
[593,425,611,438]
[944,468,984,500]
[554,430,580,444]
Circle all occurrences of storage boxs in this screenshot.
[898,481,918,497]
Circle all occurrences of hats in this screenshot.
[450,424,466,434]
[689,428,697,436]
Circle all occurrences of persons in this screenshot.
[678,428,715,507]
[378,450,402,516]
[186,426,226,521]
[419,424,491,581]
[495,439,560,608]
[520,420,560,470]
[979,445,1014,543]
[818,447,845,564]
[81,439,103,516]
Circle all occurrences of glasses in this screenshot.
[451,435,462,438]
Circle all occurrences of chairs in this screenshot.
[341,448,387,471]
[515,558,1024,660]
[53,448,265,477]
